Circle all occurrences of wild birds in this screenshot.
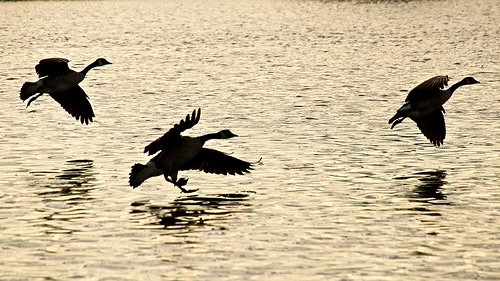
[388,73,481,147]
[127,108,264,194]
[19,58,113,125]
